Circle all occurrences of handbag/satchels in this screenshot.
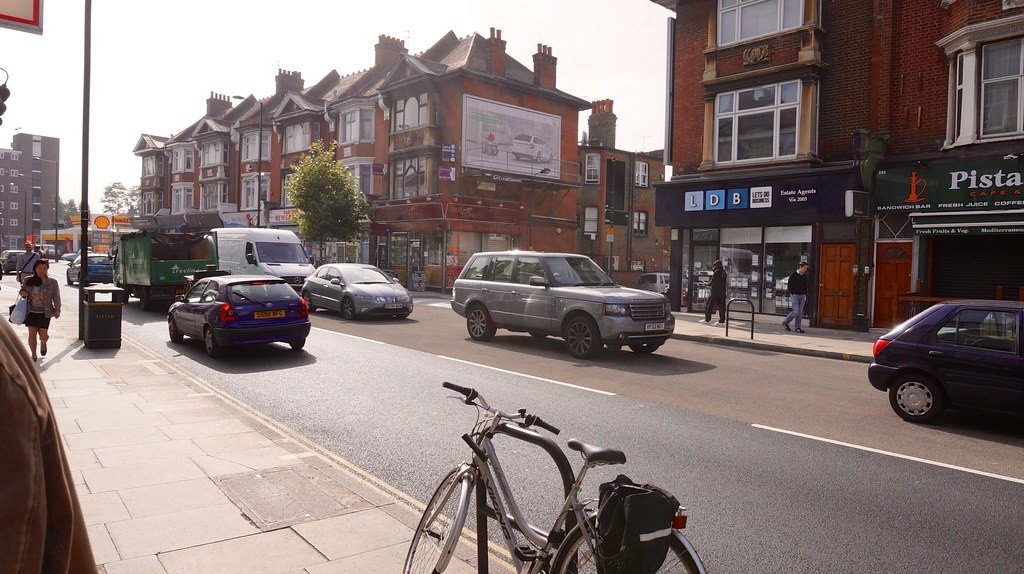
[9,289,30,325]
[594,474,679,574]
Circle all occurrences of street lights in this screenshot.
[233,95,263,227]
[10,157,58,262]
[528,169,551,250]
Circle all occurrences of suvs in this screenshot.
[449,248,675,358]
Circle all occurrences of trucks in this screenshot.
[103,228,218,310]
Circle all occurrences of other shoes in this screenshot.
[782,322,791,331]
[795,328,804,333]
[714,321,725,327]
[41,342,47,356]
[699,319,710,324]
[32,355,37,362]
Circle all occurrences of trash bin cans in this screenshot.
[83,286,123,349]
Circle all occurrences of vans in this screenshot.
[209,227,316,295]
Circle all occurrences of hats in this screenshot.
[713,260,722,266]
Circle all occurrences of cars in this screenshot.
[0,250,27,275]
[637,270,670,294]
[513,134,554,164]
[168,274,312,359]
[38,243,91,260]
[299,262,413,320]
[66,254,114,286]
[867,299,1024,423]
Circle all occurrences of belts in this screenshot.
[23,271,34,274]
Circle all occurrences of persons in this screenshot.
[550,264,576,284]
[16,241,50,284]
[252,243,265,260]
[331,251,340,264]
[20,260,61,362]
[284,248,298,262]
[699,260,727,327]
[782,261,809,333]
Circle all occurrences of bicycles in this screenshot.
[404,382,707,574]
